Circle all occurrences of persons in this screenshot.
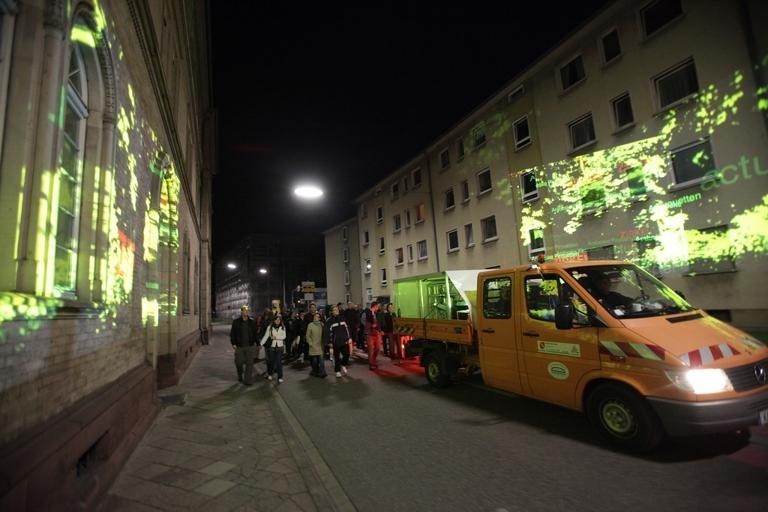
[595,278,634,310]
[228,304,260,385]
[256,299,400,382]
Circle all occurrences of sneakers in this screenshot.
[263,364,349,383]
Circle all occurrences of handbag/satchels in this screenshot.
[263,323,274,349]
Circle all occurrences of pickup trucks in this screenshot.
[393,255,768,440]
